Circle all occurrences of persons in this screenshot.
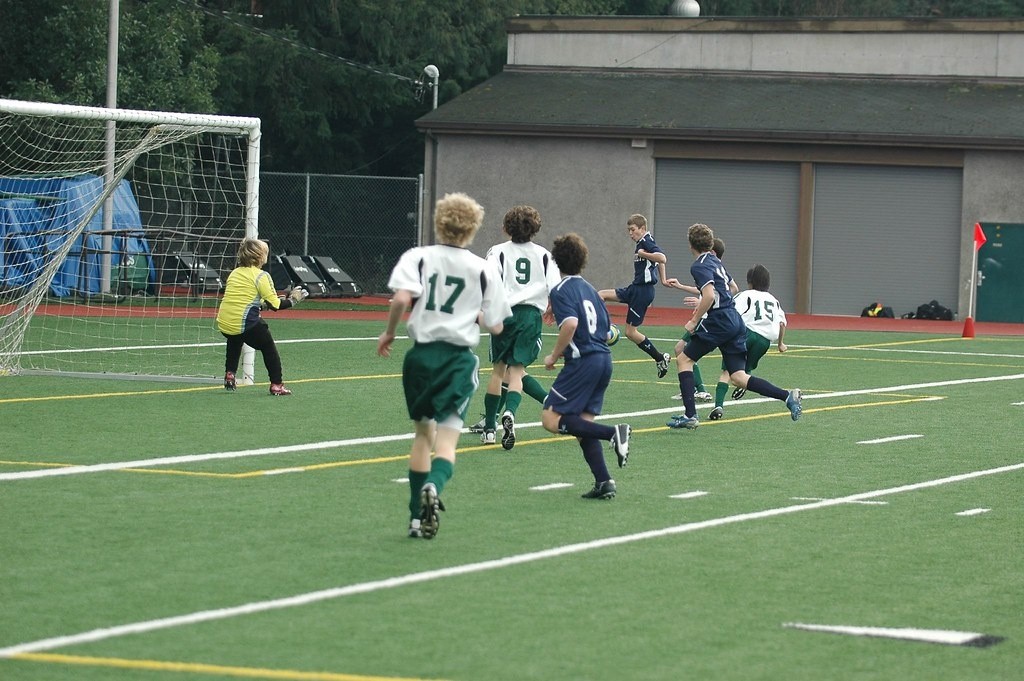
[469,205,562,450]
[216,237,309,395]
[666,224,802,430]
[708,265,787,420]
[542,232,632,499]
[597,214,674,378]
[377,192,513,539]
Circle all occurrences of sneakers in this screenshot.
[785,388,804,421]
[656,352,671,378]
[501,410,516,450]
[666,414,699,429]
[707,406,723,420]
[420,483,440,540]
[408,519,422,537]
[697,392,713,400]
[224,370,236,391]
[480,426,497,443]
[469,412,498,433]
[609,423,632,469]
[671,392,696,400]
[581,479,616,499]
[732,386,746,400]
[270,383,291,396]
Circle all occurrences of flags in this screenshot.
[973,223,987,251]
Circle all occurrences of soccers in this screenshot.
[606,324,621,346]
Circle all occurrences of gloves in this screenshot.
[286,286,309,307]
